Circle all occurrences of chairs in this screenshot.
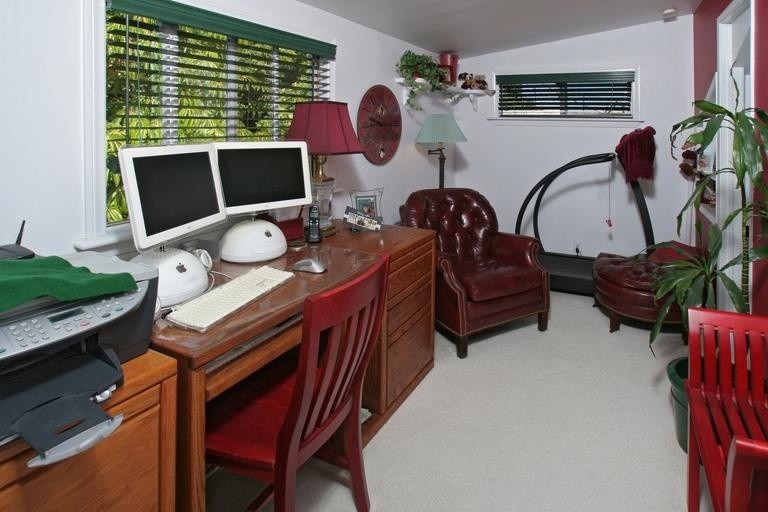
[683,306,768,512]
[205,251,391,512]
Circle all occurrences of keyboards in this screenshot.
[166,264,296,334]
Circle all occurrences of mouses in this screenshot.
[293,258,326,273]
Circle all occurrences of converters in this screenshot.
[1,221,35,262]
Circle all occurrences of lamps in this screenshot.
[284,101,364,239]
[415,113,467,188]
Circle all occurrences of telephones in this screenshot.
[306,205,322,243]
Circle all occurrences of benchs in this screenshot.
[593,252,707,333]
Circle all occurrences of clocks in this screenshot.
[357,86,402,165]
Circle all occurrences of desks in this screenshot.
[150,219,437,512]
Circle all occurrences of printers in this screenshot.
[0,248,160,469]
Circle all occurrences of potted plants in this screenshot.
[637,60,768,455]
[400,50,454,110]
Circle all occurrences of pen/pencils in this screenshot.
[298,205,304,218]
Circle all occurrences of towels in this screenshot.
[0,256,138,312]
[617,126,657,183]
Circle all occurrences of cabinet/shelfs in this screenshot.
[1,348,177,512]
[693,72,718,229]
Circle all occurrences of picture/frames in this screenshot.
[341,188,385,234]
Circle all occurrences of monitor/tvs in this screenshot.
[117,144,227,310]
[212,141,312,263]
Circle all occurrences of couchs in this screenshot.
[399,187,549,359]
